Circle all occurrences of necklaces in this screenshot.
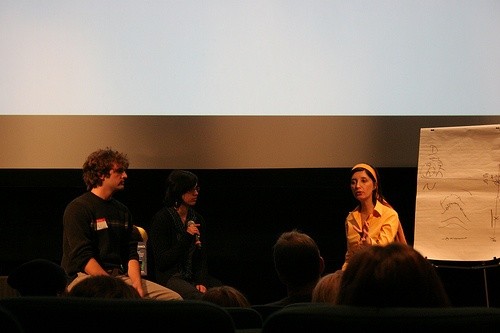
[360,205,375,218]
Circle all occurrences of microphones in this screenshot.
[187,220,202,250]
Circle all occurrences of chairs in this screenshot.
[0,292,500,333]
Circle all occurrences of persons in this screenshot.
[148,169,206,300]
[59,146,182,300]
[269,231,324,304]
[313,269,344,303]
[338,242,455,305]
[197,285,250,306]
[65,275,144,300]
[342,161,407,273]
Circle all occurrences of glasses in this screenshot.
[188,187,201,192]
